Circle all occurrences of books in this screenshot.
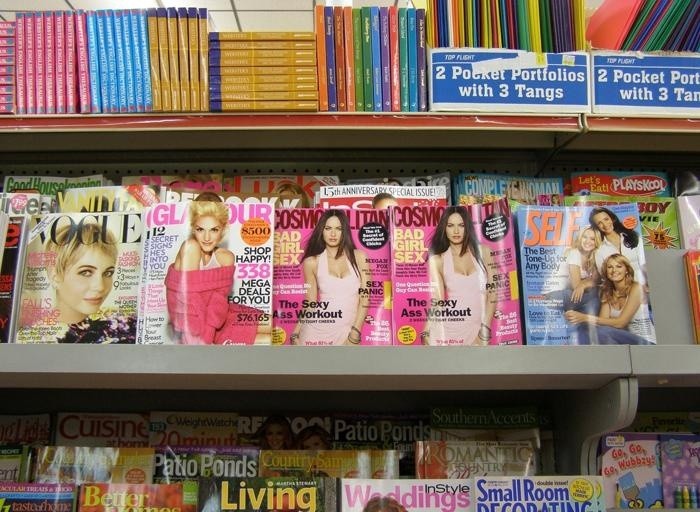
[1,171,700,346]
[1,411,700,512]
[1,1,700,115]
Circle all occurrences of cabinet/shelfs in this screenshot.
[0,115,700,475]
